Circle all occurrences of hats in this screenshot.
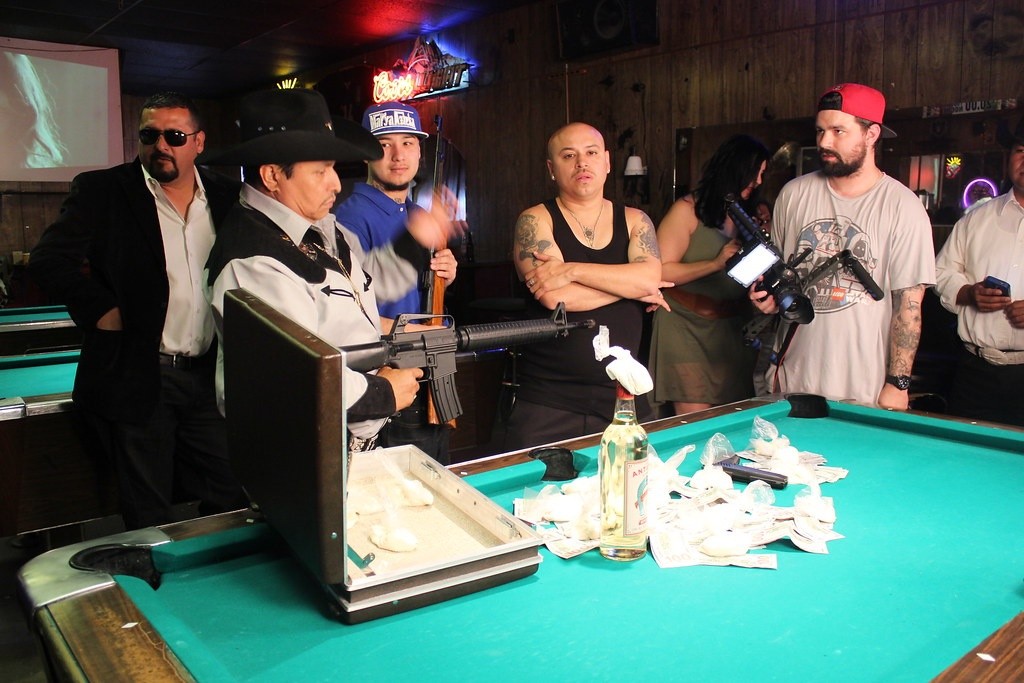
[995,117,1024,146]
[817,82,897,138]
[202,89,384,167]
[363,100,430,138]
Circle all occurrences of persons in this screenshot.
[334,102,457,467]
[28,90,248,533]
[648,132,771,416]
[204,79,457,500]
[0,50,66,170]
[930,131,1024,427]
[507,121,674,453]
[749,83,937,412]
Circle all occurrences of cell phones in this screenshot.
[985,276,1011,297]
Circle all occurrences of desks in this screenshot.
[0,305,77,344]
[0,344,79,424]
[12,388,1023,683]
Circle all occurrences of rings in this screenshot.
[658,290,661,296]
[530,278,535,287]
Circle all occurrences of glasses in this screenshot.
[139,127,203,147]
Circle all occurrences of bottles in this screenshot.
[599,380,649,562]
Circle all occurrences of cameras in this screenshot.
[725,235,816,325]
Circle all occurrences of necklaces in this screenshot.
[559,195,604,247]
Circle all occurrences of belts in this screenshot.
[153,355,207,368]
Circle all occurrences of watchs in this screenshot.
[885,373,912,391]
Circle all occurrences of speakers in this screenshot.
[555,0,661,62]
